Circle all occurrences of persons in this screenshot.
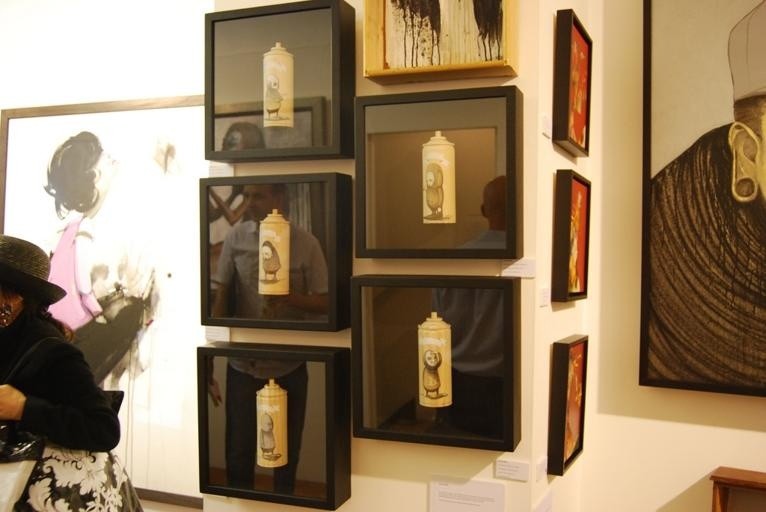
[0,233,143,511]
[206,119,292,277]
[206,180,332,499]
[426,174,510,440]
[39,128,113,334]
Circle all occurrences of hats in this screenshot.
[0,236,67,305]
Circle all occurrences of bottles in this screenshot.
[255,379,289,468]
[421,129,458,225]
[258,208,292,297]
[418,310,455,409]
[262,41,296,129]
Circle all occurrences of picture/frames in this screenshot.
[1,96,203,507]
[637,1,766,396]
[547,332,591,475]
[550,167,592,304]
[554,7,594,159]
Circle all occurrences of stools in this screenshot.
[710,465,766,511]
